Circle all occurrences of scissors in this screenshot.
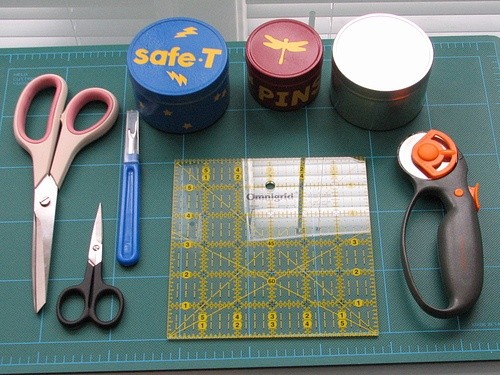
[11,74,119,314]
[55,202,124,329]
